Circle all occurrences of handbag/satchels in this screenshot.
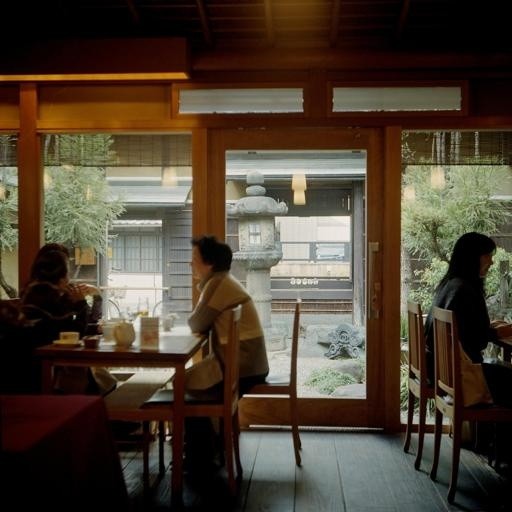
[459,343,492,407]
[184,352,223,391]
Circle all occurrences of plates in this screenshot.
[52,340,82,347]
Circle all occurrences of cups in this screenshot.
[59,332,80,343]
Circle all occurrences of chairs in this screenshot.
[429,306,512,503]
[403,301,452,472]
[244,300,302,467]
[140,305,242,496]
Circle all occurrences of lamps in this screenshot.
[291,174,309,207]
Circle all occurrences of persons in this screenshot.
[424,231,511,409]
[17,242,118,395]
[10,251,87,394]
[167,234,270,471]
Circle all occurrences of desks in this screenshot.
[1,394,131,511]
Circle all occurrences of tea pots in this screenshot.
[113,318,136,347]
